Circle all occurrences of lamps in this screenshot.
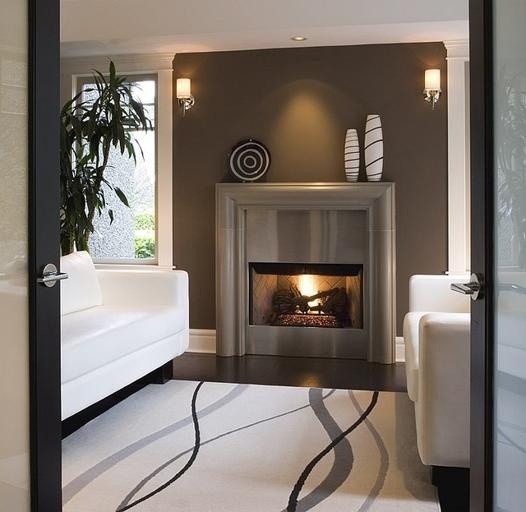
[175,77,195,119]
[422,68,442,108]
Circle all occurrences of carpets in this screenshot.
[62,379,442,512]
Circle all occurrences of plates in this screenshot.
[226,137,271,182]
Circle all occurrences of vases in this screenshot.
[342,113,384,183]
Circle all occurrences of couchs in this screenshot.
[58,249,189,423]
[402,273,471,488]
